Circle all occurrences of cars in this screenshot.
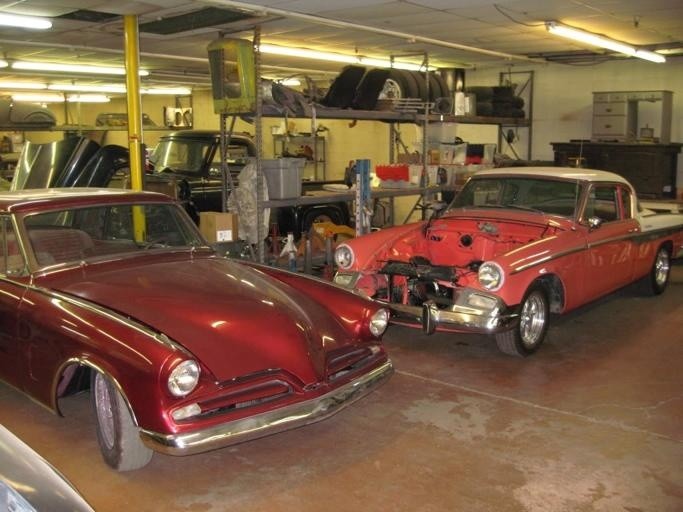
[0,187,395,473]
[329,166,683,358]
[150,129,352,241]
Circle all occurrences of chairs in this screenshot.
[27,229,98,268]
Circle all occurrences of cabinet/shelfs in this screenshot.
[548,141,683,200]
[226,26,428,262]
[274,133,326,182]
[427,54,534,122]
[591,90,674,143]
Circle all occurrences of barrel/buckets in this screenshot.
[463,93,476,116]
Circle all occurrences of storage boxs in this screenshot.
[200,211,238,245]
[429,121,497,190]
[258,157,307,200]
[376,164,423,189]
